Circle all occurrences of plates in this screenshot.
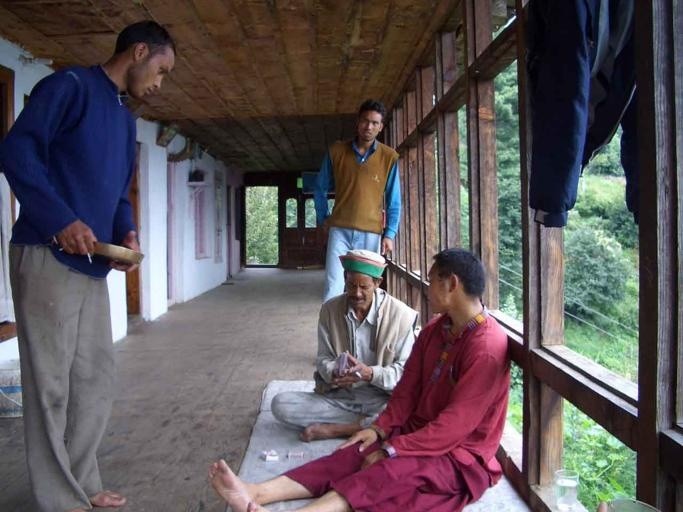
[92,240,145,264]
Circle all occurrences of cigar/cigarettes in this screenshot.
[87,251,94,267]
[356,372,364,381]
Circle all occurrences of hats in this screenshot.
[336,249,390,278]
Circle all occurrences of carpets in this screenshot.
[225,380,531,512]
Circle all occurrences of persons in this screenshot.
[209,247,512,512]
[1,19,179,511]
[270,248,424,443]
[315,98,406,307]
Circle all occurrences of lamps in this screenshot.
[18,53,53,66]
